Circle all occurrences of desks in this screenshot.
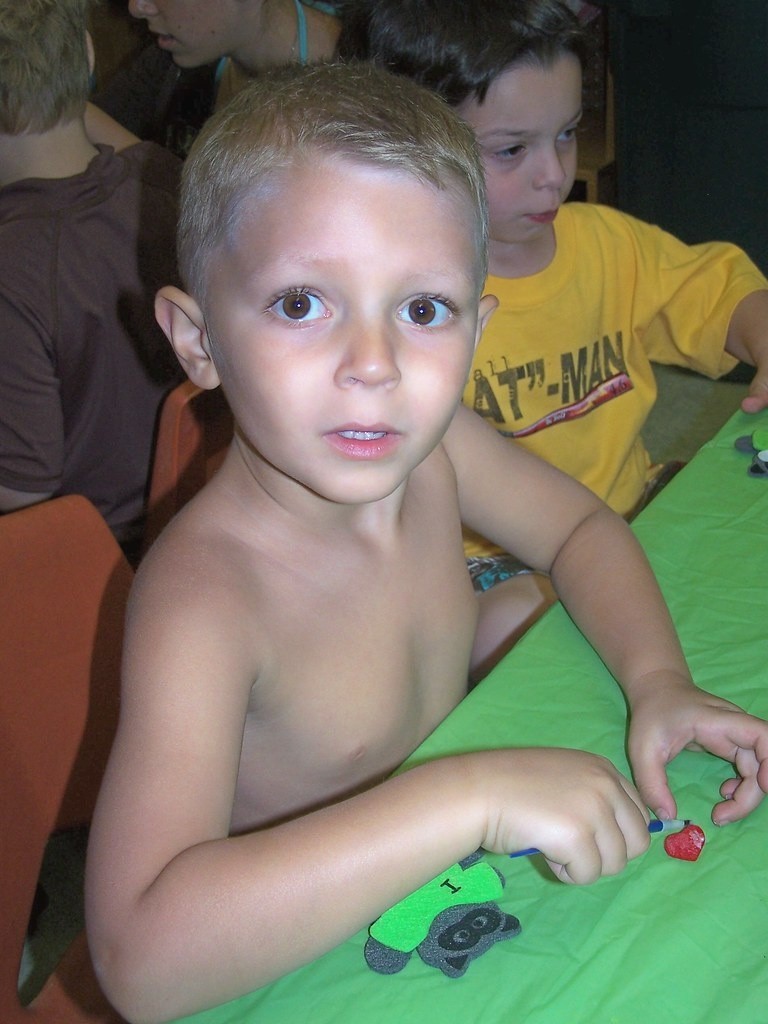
[175,410,767,1024]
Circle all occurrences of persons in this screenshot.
[0,0,185,551]
[325,0,768,675]
[85,64,768,1024]
[91,0,345,158]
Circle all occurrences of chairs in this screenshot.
[1,492,137,1023]
[138,379,234,563]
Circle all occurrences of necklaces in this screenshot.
[288,31,298,61]
